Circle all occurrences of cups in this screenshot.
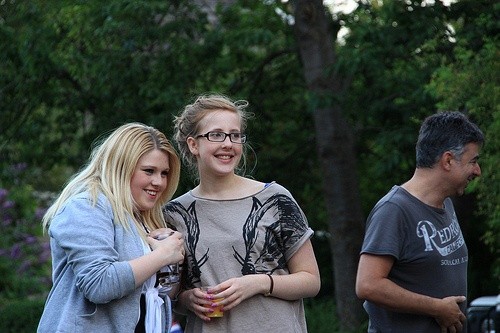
[201,286,223,318]
[149,227,180,287]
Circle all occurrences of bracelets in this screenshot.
[264,273,274,296]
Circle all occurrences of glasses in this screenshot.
[194,130,249,145]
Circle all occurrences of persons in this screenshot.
[37,121,186,333]
[161,94,320,333]
[356,111,487,333]
